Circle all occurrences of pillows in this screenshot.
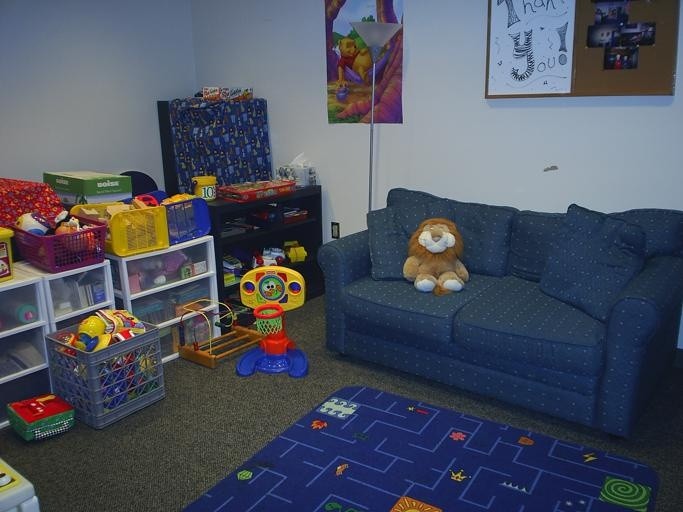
[366,199,456,282]
[538,200,651,324]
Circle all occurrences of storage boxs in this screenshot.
[40,168,133,207]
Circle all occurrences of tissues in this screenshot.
[278,151,316,188]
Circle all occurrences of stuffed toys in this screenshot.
[403,218,470,297]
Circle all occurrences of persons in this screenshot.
[622,55,632,69]
[614,54,622,69]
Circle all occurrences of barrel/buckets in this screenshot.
[191,175,217,202]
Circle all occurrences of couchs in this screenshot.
[312,184,683,445]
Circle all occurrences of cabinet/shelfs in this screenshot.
[0,267,59,432]
[101,232,223,370]
[10,258,118,344]
[155,96,324,330]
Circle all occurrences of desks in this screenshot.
[0,457,40,512]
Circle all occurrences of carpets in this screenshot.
[181,383,659,512]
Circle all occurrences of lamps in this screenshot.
[346,20,408,227]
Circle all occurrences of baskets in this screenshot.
[71,200,171,258]
[9,208,108,275]
[42,314,168,432]
[118,187,213,246]
